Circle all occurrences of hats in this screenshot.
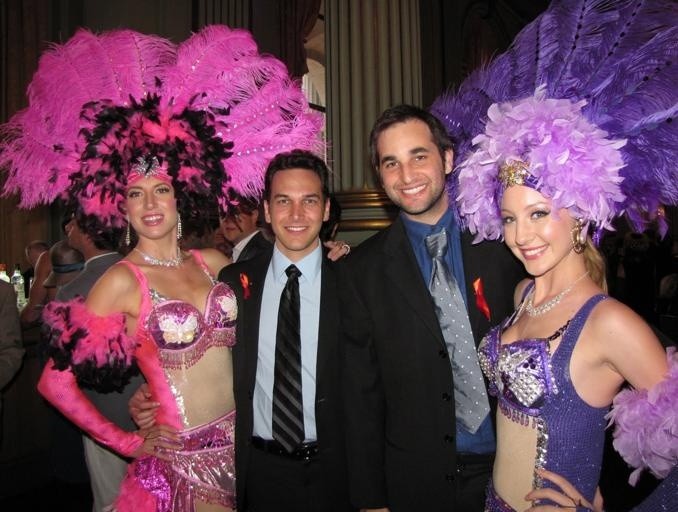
[43,240,85,288]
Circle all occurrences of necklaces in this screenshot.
[136,246,184,267]
[527,271,591,315]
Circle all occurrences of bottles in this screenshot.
[11,263,26,310]
[0,263,10,283]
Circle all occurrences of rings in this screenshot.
[342,244,350,255]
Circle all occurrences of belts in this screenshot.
[252,436,320,460]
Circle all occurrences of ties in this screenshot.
[425,228,491,435]
[272,264,304,453]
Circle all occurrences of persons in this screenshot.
[130,150,357,511]
[335,104,533,511]
[55,200,147,512]
[218,199,270,264]
[1,24,350,511]
[431,1,678,511]
[1,202,94,511]
